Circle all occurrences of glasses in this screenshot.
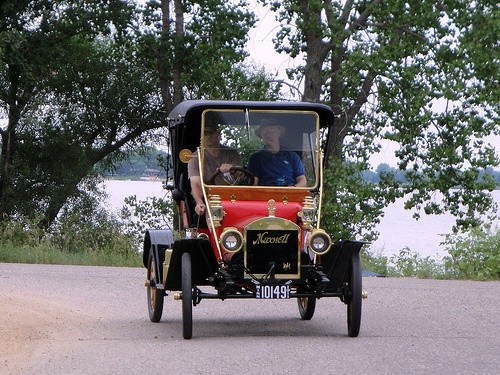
[204,129,216,136]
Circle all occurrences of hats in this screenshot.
[255,118,285,138]
[205,120,217,127]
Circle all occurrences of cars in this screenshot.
[143,100,373,340]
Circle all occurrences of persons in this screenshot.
[247,118,307,187]
[188,118,244,216]
